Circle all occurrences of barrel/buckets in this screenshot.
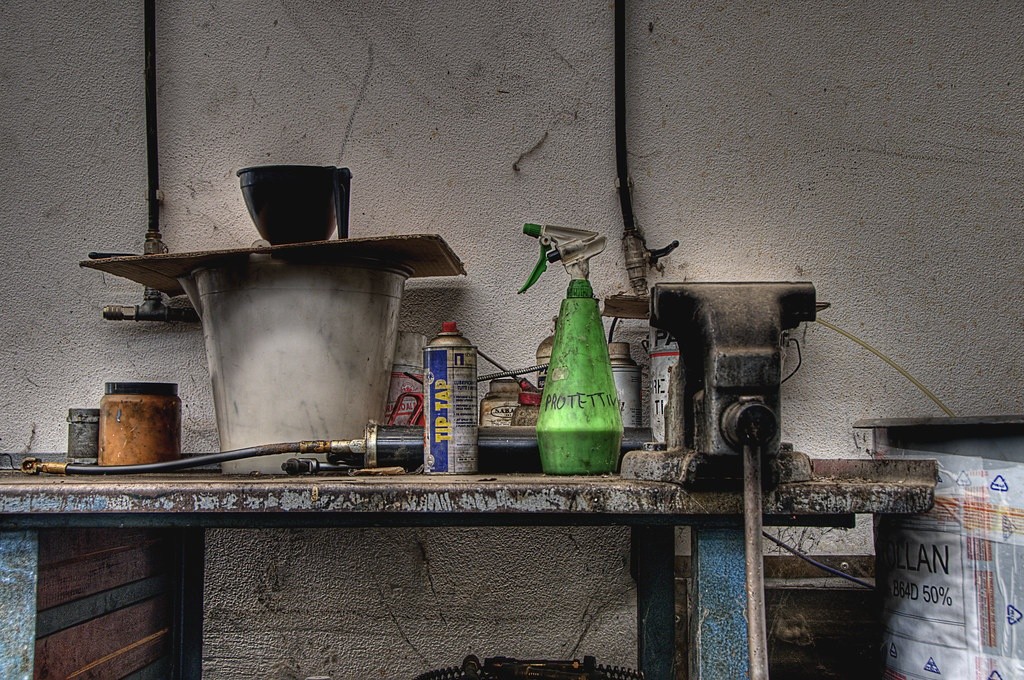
[191,263,415,476]
[67,408,99,464]
[98,382,182,467]
[648,350,680,442]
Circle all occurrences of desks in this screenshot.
[0,452,944,680]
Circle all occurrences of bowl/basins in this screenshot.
[236,165,354,245]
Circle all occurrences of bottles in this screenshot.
[422,322,479,474]
[511,391,544,427]
[608,342,643,430]
